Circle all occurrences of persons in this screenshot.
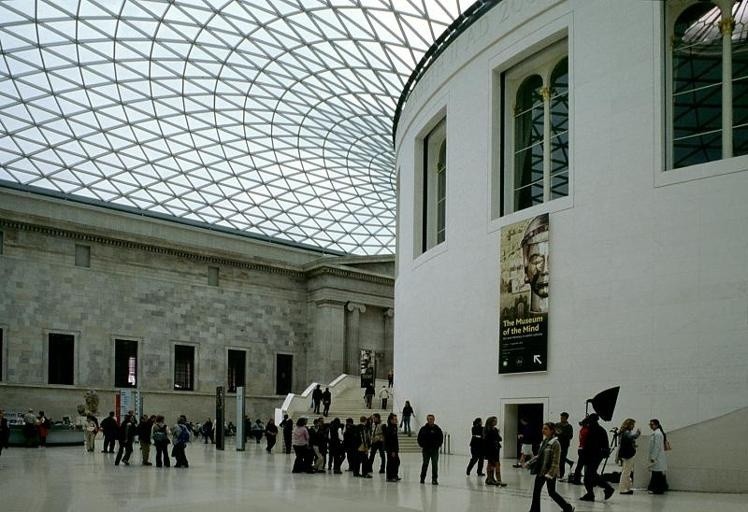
[245,368,417,482]
[466,418,486,477]
[481,415,510,488]
[227,421,235,438]
[418,414,443,485]
[514,409,669,512]
[522,216,549,313]
[82,409,218,466]
[0,408,10,459]
[18,409,49,447]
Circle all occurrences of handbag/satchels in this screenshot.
[663,432,672,451]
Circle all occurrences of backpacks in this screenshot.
[153,423,167,443]
[177,424,190,444]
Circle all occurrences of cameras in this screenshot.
[610,427,618,432]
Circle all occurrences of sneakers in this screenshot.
[578,488,615,502]
[620,489,634,495]
